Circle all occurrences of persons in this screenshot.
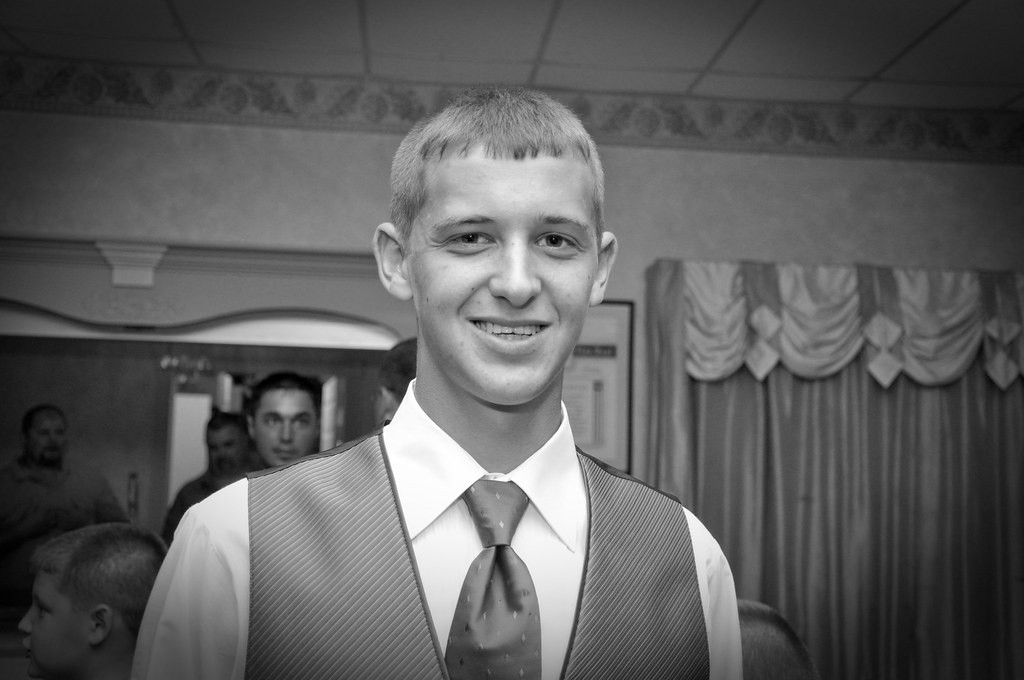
[128,83,742,680]
[16,523,169,680]
[161,413,251,550]
[0,400,130,569]
[377,335,418,425]
[244,371,322,472]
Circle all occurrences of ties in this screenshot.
[445,480,542,680]
